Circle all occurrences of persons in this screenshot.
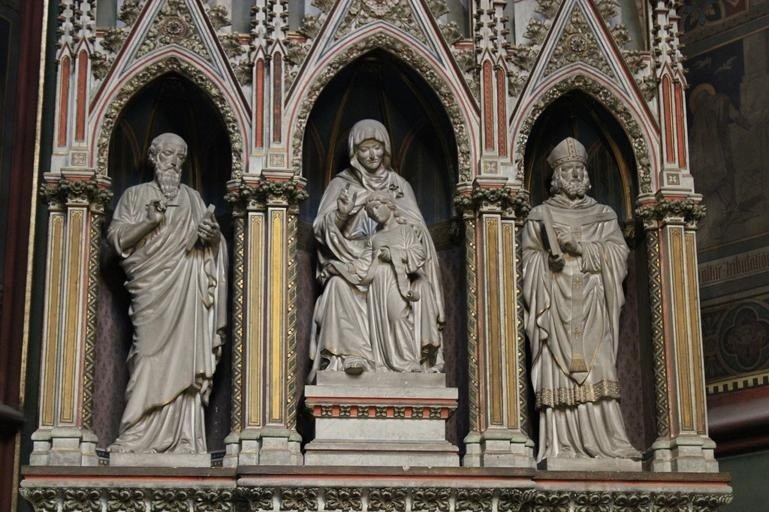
[363,194,427,373]
[106,133,228,454]
[522,137,647,462]
[314,119,446,373]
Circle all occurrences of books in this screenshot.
[540,206,560,258]
[185,203,216,252]
